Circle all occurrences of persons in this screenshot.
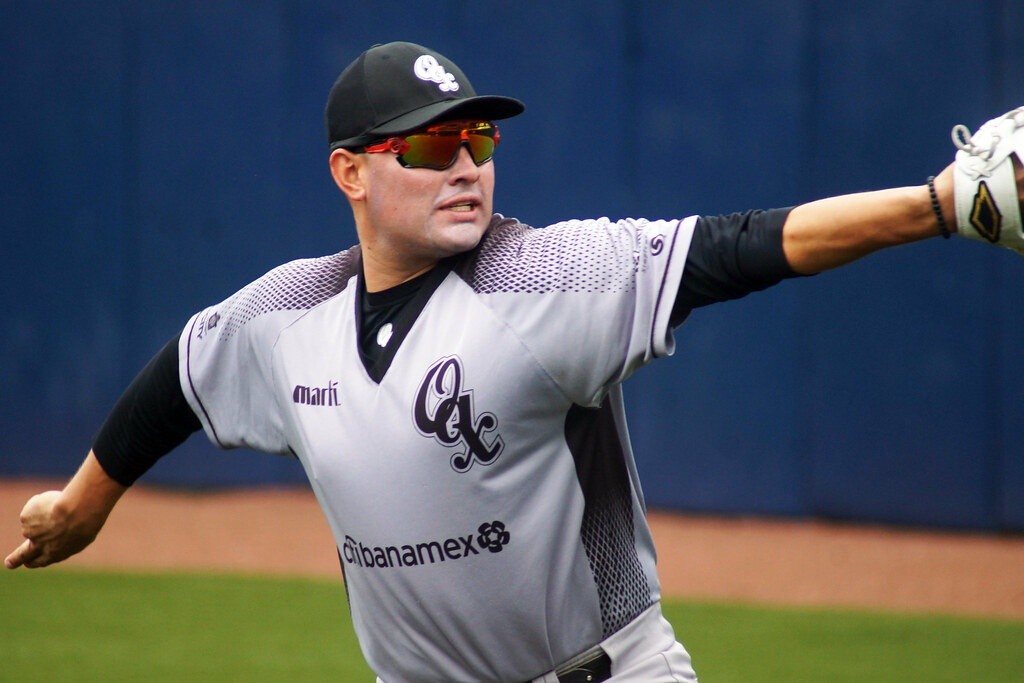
[4,40,1024,683]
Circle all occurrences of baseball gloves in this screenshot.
[950,105,1024,254]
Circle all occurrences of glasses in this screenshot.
[364,119,501,171]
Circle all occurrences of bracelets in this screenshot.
[927,175,952,241]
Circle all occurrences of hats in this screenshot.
[325,42,525,154]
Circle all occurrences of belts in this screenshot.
[557,654,611,683]
